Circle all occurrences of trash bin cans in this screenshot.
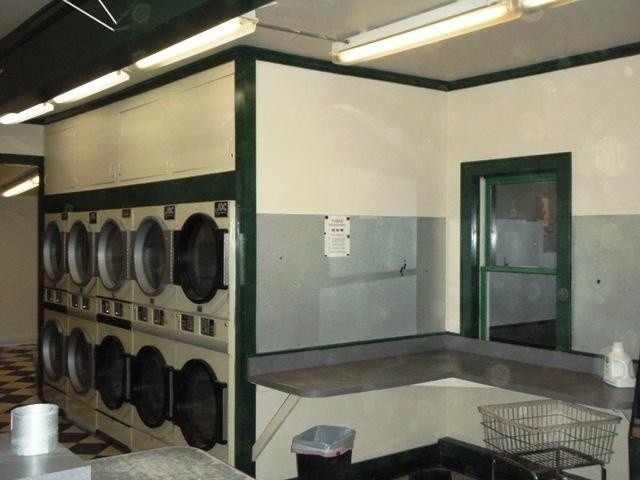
[291,424,355,480]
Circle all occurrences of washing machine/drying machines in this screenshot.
[39,202,233,468]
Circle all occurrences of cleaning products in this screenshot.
[603,341,636,388]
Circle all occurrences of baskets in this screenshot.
[477,400,621,473]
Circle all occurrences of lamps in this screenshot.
[0,169,41,199]
[518,0,577,17]
[133,10,258,90]
[326,1,521,65]
[53,64,132,121]
[0,101,54,126]
